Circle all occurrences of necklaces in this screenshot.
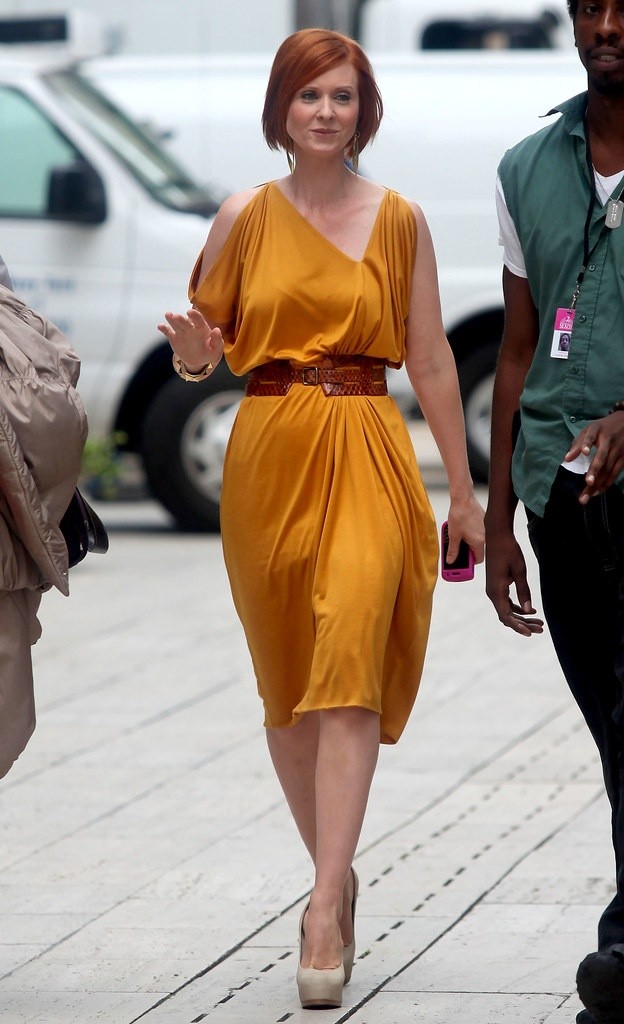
[584,106,624,229]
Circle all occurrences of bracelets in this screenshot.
[608,400,624,414]
[176,356,214,383]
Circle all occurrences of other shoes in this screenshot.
[575,950,624,1024]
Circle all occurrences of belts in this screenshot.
[244,354,389,397]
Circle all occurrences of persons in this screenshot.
[157,27,485,1009]
[0,255,88,780]
[484,0,624,1023]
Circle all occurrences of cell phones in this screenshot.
[441,520,474,582]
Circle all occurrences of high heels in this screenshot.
[295,898,346,1008]
[343,864,359,985]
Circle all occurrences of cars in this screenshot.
[2,0,622,530]
